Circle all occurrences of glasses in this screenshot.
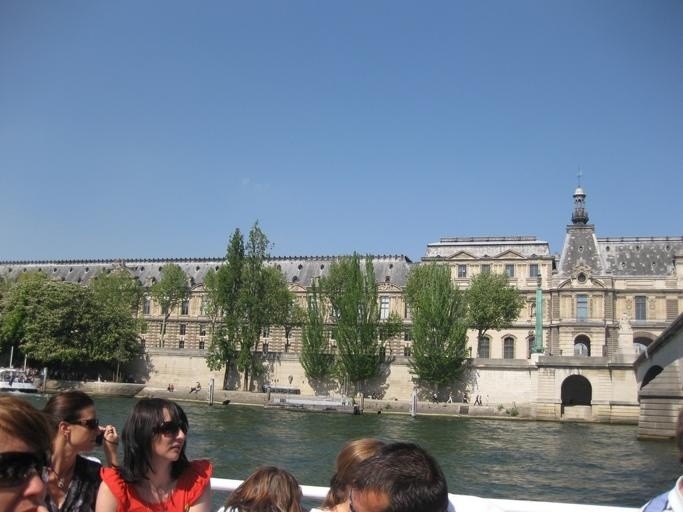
[159,422,187,435]
[0,452,47,487]
[69,419,98,429]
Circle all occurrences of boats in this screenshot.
[264,399,363,414]
[0,366,37,393]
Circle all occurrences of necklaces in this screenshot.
[53,466,75,489]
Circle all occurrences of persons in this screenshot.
[309,438,387,512]
[17,368,92,383]
[189,382,201,394]
[42,391,104,512]
[432,391,488,406]
[348,442,455,512]
[0,394,54,512]
[94,398,214,512]
[167,384,174,392]
[80,424,119,471]
[640,404,683,512]
[218,467,311,512]
